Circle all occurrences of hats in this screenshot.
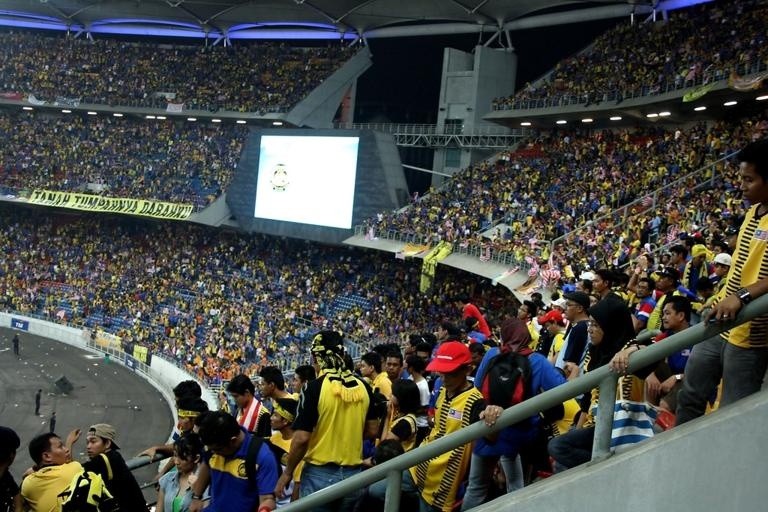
[691,244,707,258]
[426,340,472,374]
[88,424,121,451]
[724,225,739,236]
[562,291,590,310]
[714,253,732,266]
[655,267,680,280]
[537,309,564,325]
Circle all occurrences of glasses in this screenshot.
[672,253,677,256]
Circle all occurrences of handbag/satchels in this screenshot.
[591,376,657,452]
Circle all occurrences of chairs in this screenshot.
[0,101,559,419]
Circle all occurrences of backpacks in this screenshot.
[480,343,534,432]
